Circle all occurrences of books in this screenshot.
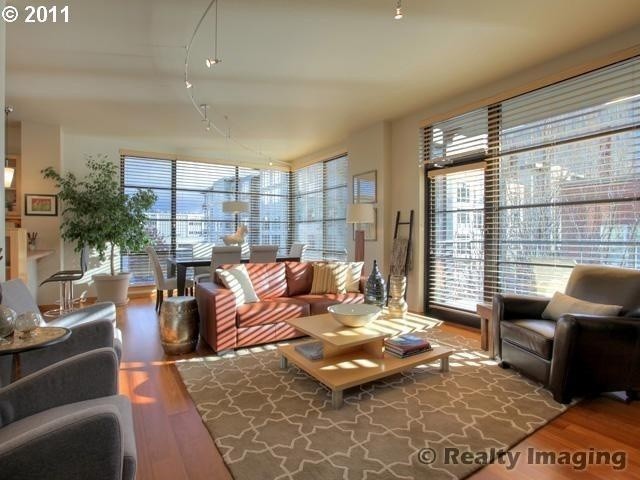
[383,348,433,359]
[385,343,430,356]
[384,334,430,352]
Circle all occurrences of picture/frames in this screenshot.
[25,194,58,216]
[352,170,377,205]
[353,208,377,241]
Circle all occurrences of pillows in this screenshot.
[327,262,364,293]
[216,263,260,307]
[309,262,348,294]
[541,291,624,322]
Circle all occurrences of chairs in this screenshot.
[145,246,193,315]
[289,243,307,262]
[193,242,216,283]
[36,245,90,318]
[250,245,279,263]
[209,246,241,282]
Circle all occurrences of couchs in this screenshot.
[0,278,122,389]
[0,346,137,480]
[195,260,368,357]
[491,263,640,405]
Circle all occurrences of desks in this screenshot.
[167,256,300,297]
[27,249,55,301]
[0,327,72,384]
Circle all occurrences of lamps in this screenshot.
[4,167,14,188]
[346,204,374,231]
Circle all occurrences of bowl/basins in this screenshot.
[327,304,380,327]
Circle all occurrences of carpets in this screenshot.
[174,328,583,480]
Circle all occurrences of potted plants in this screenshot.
[40,152,160,307]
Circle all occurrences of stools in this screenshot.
[477,301,492,351]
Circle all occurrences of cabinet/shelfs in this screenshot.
[5,153,21,228]
